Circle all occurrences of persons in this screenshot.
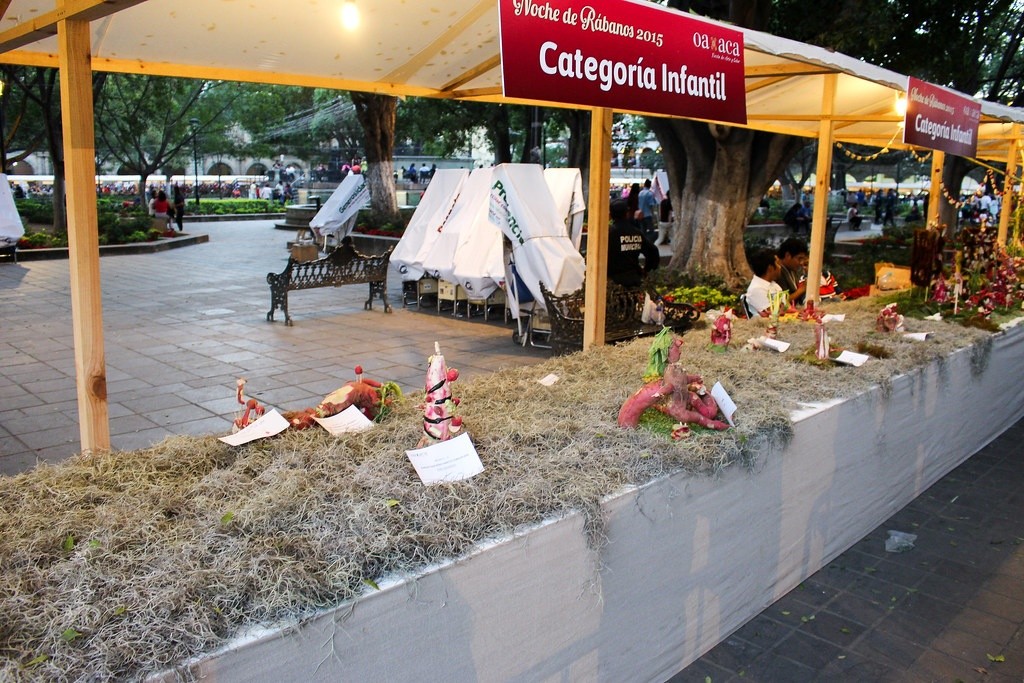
[611,148,673,248]
[760,174,929,231]
[744,236,846,316]
[148,186,185,231]
[959,171,999,222]
[10,151,437,203]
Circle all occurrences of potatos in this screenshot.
[615,334,732,432]
[711,297,902,346]
[233,341,464,445]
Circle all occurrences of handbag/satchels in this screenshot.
[634,210,643,219]
[167,207,175,218]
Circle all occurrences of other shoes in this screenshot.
[852,227,860,231]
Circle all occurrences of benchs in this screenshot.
[539,278,700,358]
[267,234,394,325]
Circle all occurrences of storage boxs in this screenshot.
[292,244,317,265]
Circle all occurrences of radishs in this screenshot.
[424,342,451,444]
